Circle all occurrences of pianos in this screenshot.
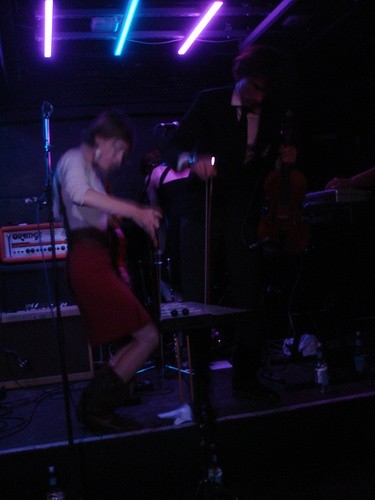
[260,189,370,213]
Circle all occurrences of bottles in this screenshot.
[47,466,67,500]
[316,344,329,397]
[206,442,225,486]
[354,330,368,381]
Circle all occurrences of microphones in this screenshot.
[41,101,54,116]
[158,120,181,128]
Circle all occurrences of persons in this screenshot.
[258,131,351,256]
[163,45,285,423]
[52,109,162,435]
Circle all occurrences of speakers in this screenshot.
[0,305,94,390]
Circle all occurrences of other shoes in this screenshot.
[77,399,142,434]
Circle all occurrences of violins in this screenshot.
[105,222,132,286]
[257,123,310,262]
